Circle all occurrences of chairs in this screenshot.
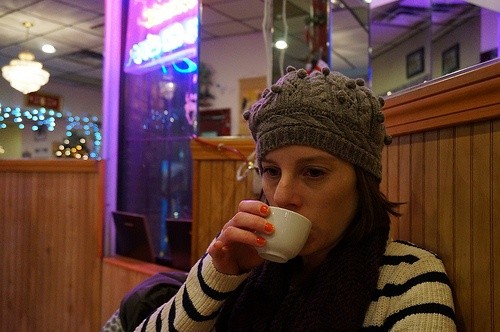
[111,211,192,266]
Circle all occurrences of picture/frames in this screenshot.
[406,46,424,77]
[442,43,460,75]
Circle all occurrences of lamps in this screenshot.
[1,21,50,95]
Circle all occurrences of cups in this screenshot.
[253,205,314,265]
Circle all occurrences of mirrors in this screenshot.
[103,0,203,281]
[272,0,481,97]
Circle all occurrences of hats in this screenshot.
[243,66,392,183]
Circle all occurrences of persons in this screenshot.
[63,128,88,158]
[132,66,459,332]
